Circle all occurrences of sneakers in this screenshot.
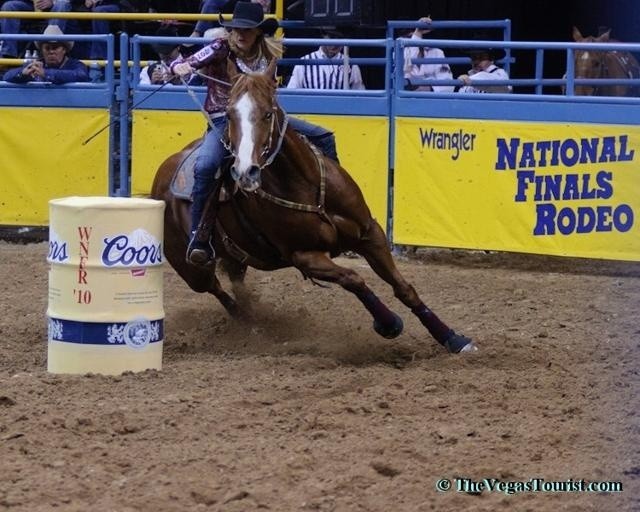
[189,250,207,264]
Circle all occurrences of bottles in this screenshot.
[23,49,32,68]
[32,50,39,63]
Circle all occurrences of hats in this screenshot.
[152,27,180,54]
[218,2,278,36]
[464,29,505,60]
[34,25,73,52]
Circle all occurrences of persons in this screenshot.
[168,1,338,267]
[455,48,514,94]
[393,15,455,93]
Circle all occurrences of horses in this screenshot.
[150,55,478,353]
